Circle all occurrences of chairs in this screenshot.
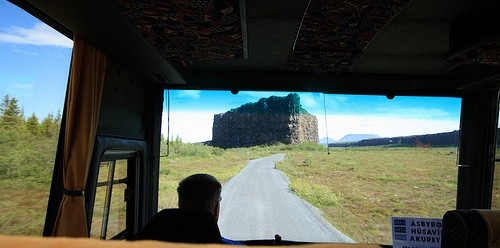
[133,208,224,244]
[439,209,500,248]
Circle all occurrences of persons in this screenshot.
[176,174,245,245]
